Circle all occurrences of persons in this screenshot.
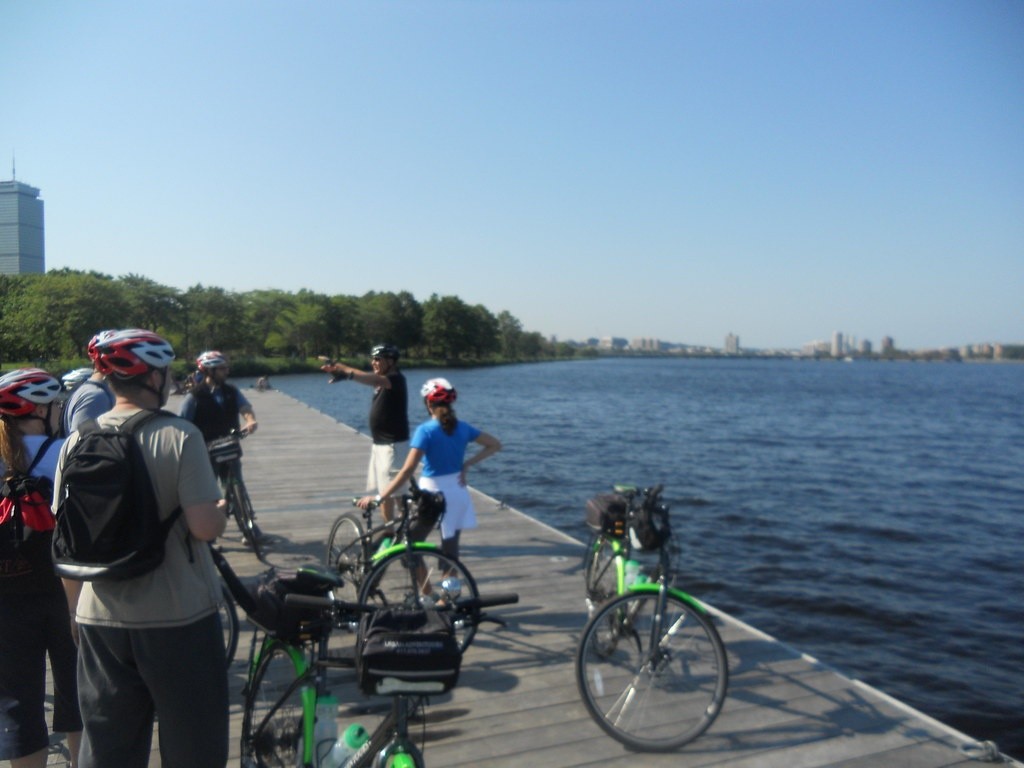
[191,364,203,384]
[356,378,499,605]
[51,328,231,767]
[257,373,270,389]
[179,352,257,550]
[0,368,83,767]
[62,331,118,438]
[321,345,410,552]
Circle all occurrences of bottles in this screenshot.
[624,559,647,606]
[321,722,369,767]
[311,691,339,768]
[369,536,392,568]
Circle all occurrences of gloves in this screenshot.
[317,354,356,384]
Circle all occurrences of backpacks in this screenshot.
[51,408,185,583]
[0,438,55,575]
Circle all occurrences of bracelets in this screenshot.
[375,495,381,504]
[349,371,354,379]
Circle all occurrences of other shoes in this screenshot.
[417,592,439,609]
[436,589,462,606]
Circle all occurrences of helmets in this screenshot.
[372,344,401,363]
[420,378,457,403]
[197,352,232,376]
[0,370,60,419]
[88,329,174,378]
[61,367,94,389]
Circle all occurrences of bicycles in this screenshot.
[322,468,481,657]
[237,565,521,768]
[574,477,730,753]
[206,427,266,560]
